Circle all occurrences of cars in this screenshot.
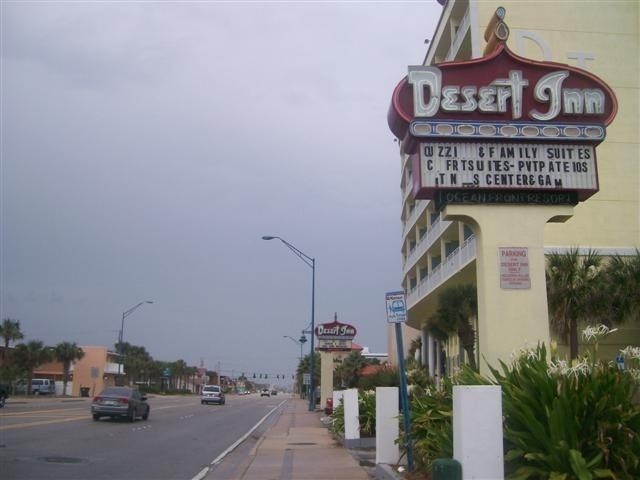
[90,388,150,421]
[0,385,9,408]
[271,389,277,396]
[260,388,270,397]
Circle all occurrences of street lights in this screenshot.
[260,234,317,412]
[281,333,303,398]
[117,299,154,385]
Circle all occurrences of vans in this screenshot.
[201,385,226,404]
[10,378,56,396]
